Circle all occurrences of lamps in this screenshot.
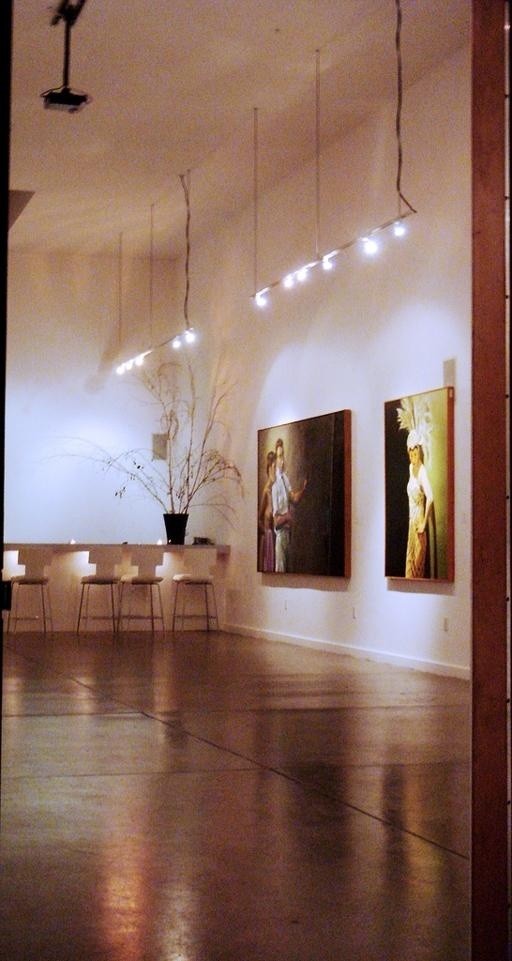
[114,169,196,375]
[249,0,417,306]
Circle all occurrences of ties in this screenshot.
[281,477,291,513]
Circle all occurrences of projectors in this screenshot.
[43,86,93,115]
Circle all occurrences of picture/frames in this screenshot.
[384,387,455,583]
[257,410,352,578]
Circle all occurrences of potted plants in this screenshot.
[51,343,248,545]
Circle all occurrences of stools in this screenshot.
[171,574,220,636]
[7,576,55,639]
[76,575,121,637]
[116,575,166,644]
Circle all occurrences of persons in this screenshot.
[403,428,435,579]
[258,451,276,572]
[271,439,309,574]
